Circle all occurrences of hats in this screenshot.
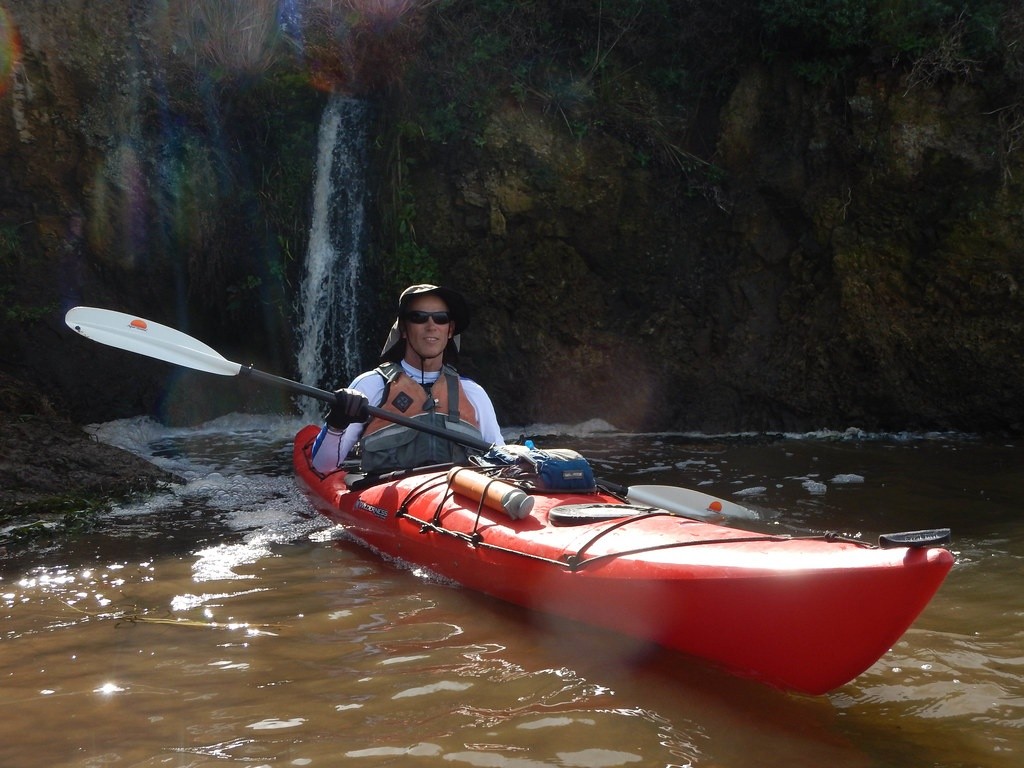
[379,284,465,363]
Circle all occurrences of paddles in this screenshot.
[63,306,760,525]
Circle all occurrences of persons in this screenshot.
[308,283,510,476]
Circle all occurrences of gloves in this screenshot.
[321,388,369,425]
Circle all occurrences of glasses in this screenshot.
[401,310,453,325]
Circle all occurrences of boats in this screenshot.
[293,425,956,700]
